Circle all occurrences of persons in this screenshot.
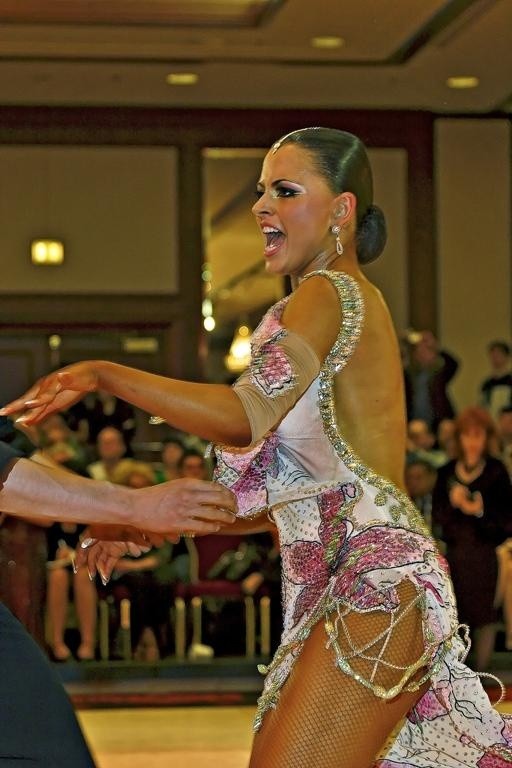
[0,413,240,767]
[1,127,511,766]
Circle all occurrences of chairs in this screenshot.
[100,534,273,664]
